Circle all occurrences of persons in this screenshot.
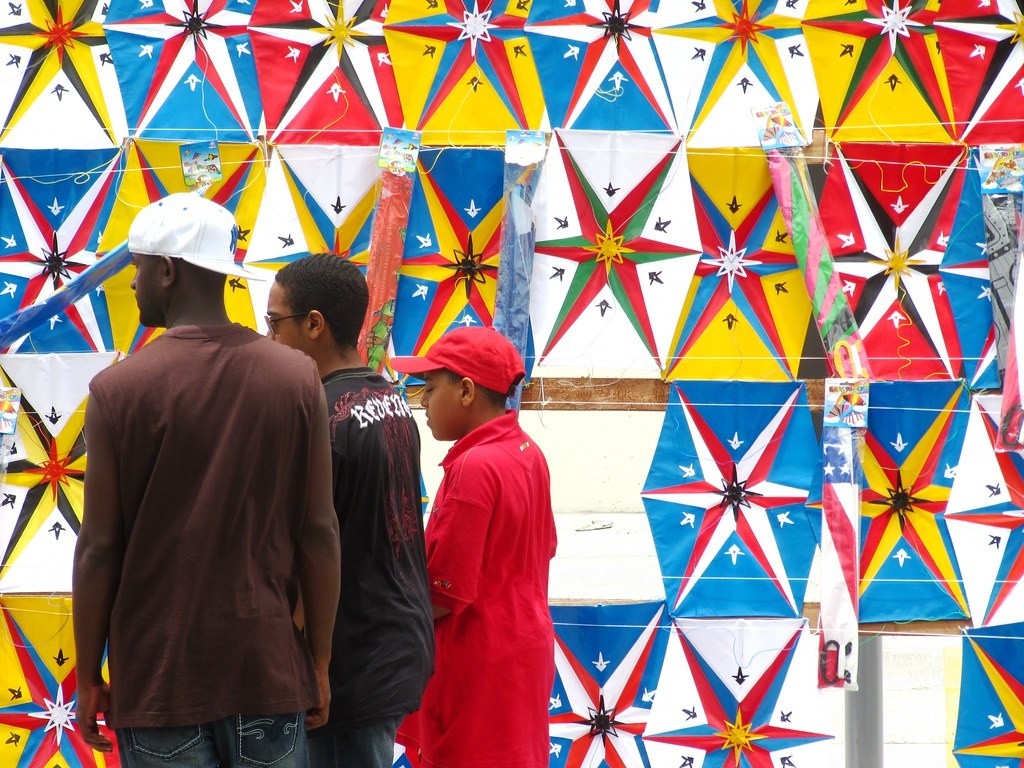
[72,191,340,768]
[261,252,436,768]
[391,325,558,767]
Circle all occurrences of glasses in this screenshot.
[264,309,343,335]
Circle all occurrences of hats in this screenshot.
[128,191,266,281]
[389,325,527,398]
